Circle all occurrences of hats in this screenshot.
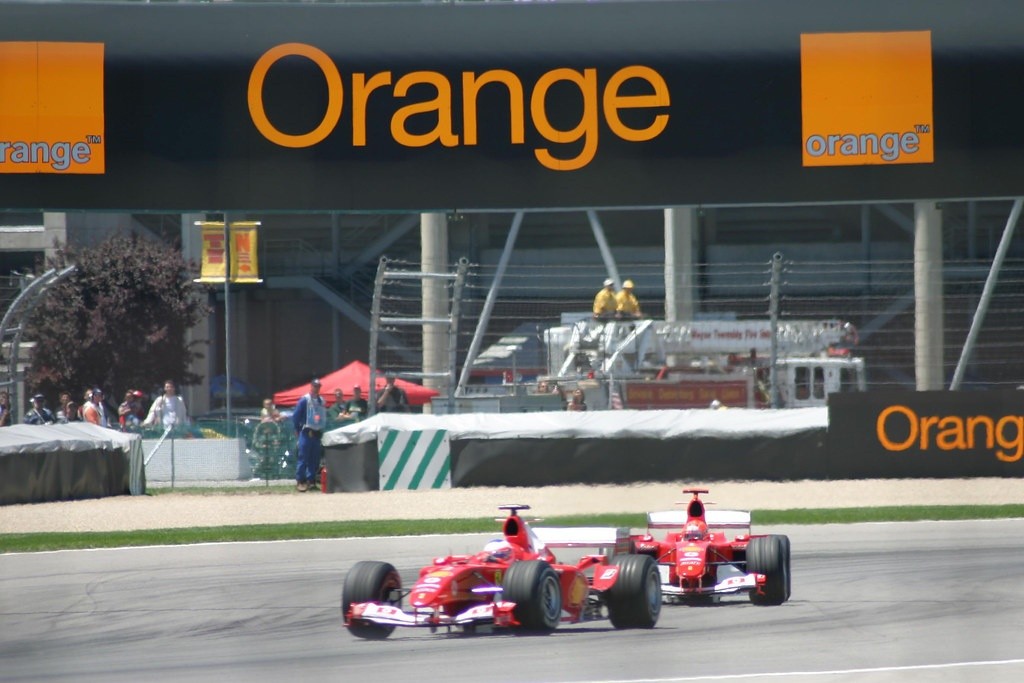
[311,378,321,387]
[602,279,613,287]
[621,280,634,289]
[353,385,360,391]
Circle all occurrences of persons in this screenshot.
[260,397,280,426]
[563,389,588,411]
[328,388,349,426]
[345,385,369,421]
[614,279,641,319]
[292,380,328,490]
[376,370,412,413]
[681,519,709,546]
[1,380,186,432]
[479,539,514,565]
[591,278,619,318]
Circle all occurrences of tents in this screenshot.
[273,356,440,416]
